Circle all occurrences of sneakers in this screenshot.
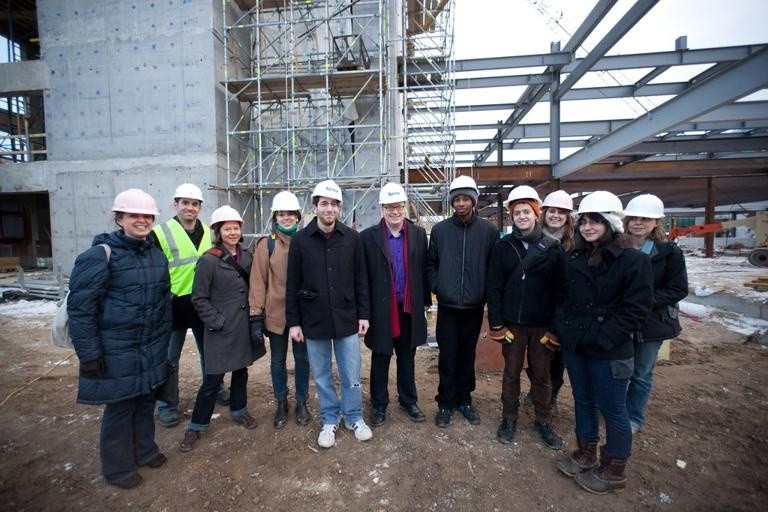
[436,405,456,428]
[457,403,481,425]
[317,421,339,448]
[343,415,373,442]
[231,411,258,429]
[180,429,203,452]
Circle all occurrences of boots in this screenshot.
[556,433,600,477]
[524,367,537,406]
[575,444,632,494]
[550,377,564,416]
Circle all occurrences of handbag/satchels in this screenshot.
[51,287,75,350]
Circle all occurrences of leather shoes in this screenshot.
[368,401,389,427]
[156,402,179,427]
[136,453,166,468]
[273,397,290,428]
[110,473,144,490]
[496,417,520,443]
[397,399,426,423]
[533,420,564,450]
[292,398,311,424]
[216,389,232,405]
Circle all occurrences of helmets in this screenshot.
[208,205,245,229]
[542,189,574,211]
[504,185,543,208]
[378,182,408,205]
[311,179,344,204]
[111,188,161,216]
[172,182,204,205]
[270,190,301,213]
[623,193,665,218]
[449,174,480,195]
[577,190,625,216]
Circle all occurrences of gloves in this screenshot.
[539,331,562,354]
[489,326,515,345]
[81,358,107,379]
[248,315,270,347]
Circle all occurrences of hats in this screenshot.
[448,189,478,208]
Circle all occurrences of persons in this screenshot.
[146,183,231,428]
[486,184,565,451]
[248,191,310,429]
[622,193,688,435]
[525,190,576,417]
[285,180,373,448]
[424,175,500,428]
[179,205,267,452]
[359,182,432,426]
[67,188,170,489]
[553,190,654,495]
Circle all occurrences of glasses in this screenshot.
[316,201,338,207]
[384,206,405,211]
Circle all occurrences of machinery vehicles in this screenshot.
[670,211,766,267]
